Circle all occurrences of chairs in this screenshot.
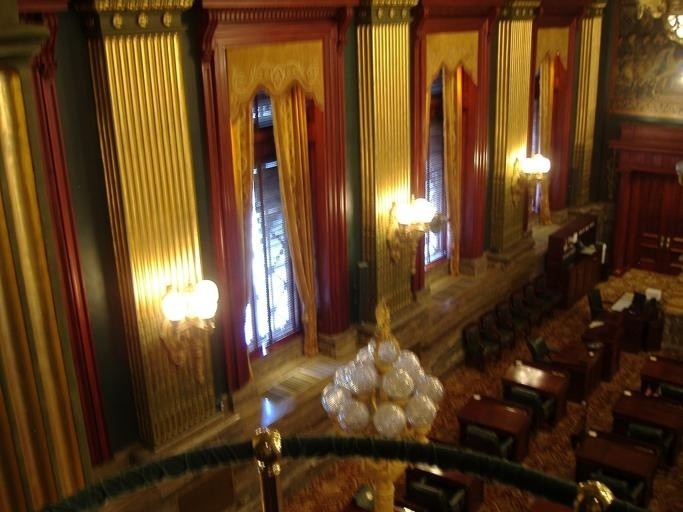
[522,212,683,512]
[456,291,567,462]
[394,467,484,511]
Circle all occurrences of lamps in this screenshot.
[509,149,551,215]
[319,296,446,511]
[383,195,437,276]
[158,274,220,385]
